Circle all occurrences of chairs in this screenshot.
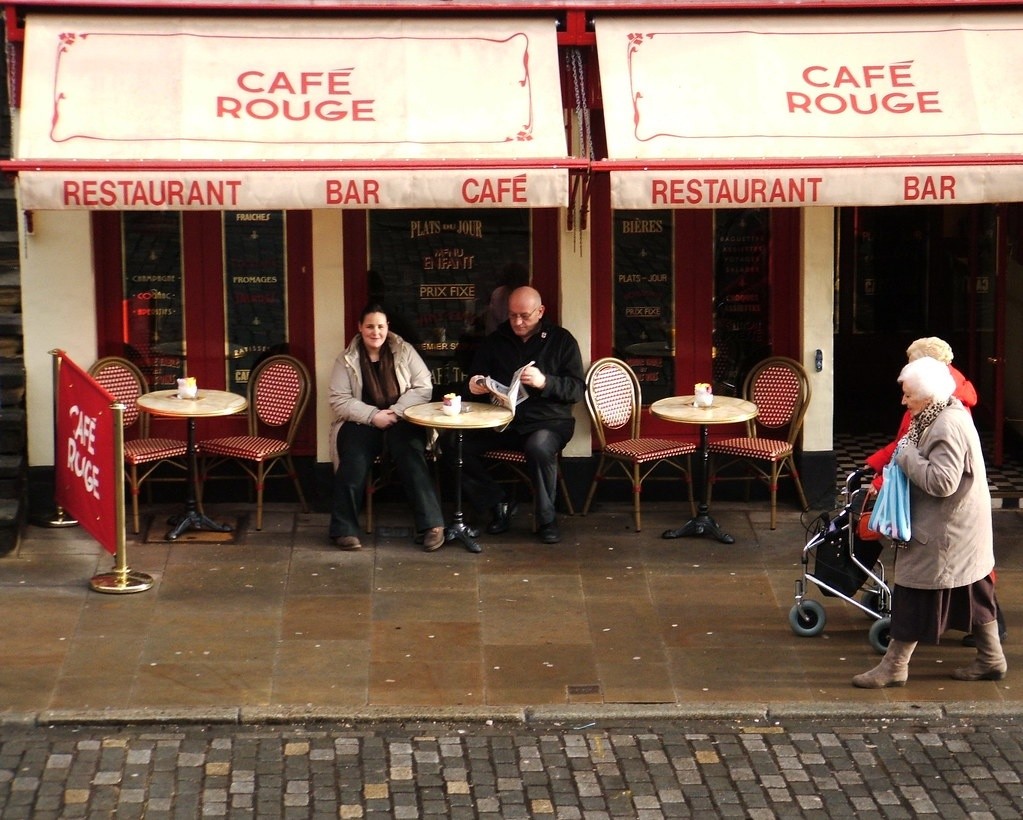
[580,357,697,534]
[707,356,812,530]
[366,439,443,534]
[464,442,574,534]
[87,356,205,535]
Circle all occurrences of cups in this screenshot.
[177,377,197,399]
[694,384,712,407]
[444,395,461,416]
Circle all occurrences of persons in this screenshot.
[461,284,587,545]
[863,336,1006,645]
[485,263,529,339]
[850,356,1007,689]
[329,303,446,551]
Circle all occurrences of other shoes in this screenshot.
[334,535,362,550]
[424,527,445,551]
[963,632,1007,646]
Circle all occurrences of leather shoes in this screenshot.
[539,517,560,542]
[488,497,518,533]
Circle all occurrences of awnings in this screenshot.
[0,17,590,209]
[594,10,1023,209]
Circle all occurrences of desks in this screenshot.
[649,395,758,544]
[403,402,513,553]
[198,354,313,531]
[136,389,247,542]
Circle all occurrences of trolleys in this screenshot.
[789,461,902,658]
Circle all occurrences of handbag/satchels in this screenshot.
[867,448,911,542]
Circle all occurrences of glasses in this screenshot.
[509,307,537,320]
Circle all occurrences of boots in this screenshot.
[851,638,919,686]
[951,619,1007,681]
